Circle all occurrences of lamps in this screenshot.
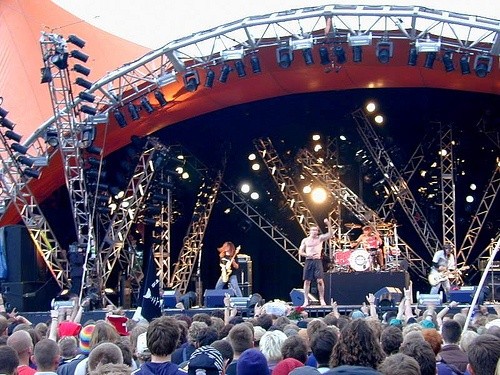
[0,32,493,309]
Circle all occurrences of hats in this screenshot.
[136,332,152,356]
[423,309,438,321]
[59,322,82,337]
[352,311,367,320]
[79,325,95,356]
[57,335,78,360]
[188,346,223,375]
[382,311,397,324]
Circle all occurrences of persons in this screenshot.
[352,226,385,269]
[429,243,455,302]
[298,220,334,307]
[0,286,500,375]
[215,242,242,297]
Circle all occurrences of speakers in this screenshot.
[159,290,181,307]
[204,288,234,309]
[373,287,404,307]
[481,272,500,302]
[290,288,320,306]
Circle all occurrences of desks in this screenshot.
[322,272,410,305]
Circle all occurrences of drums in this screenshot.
[376,229,389,237]
[333,249,372,271]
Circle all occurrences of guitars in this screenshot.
[222,247,240,282]
[428,266,469,286]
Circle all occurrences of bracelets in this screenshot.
[370,301,374,305]
[405,298,412,301]
[224,305,229,308]
[51,316,58,319]
[446,304,452,310]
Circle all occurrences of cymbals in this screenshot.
[332,238,348,243]
[361,210,374,223]
[344,223,361,229]
[375,224,393,227]
